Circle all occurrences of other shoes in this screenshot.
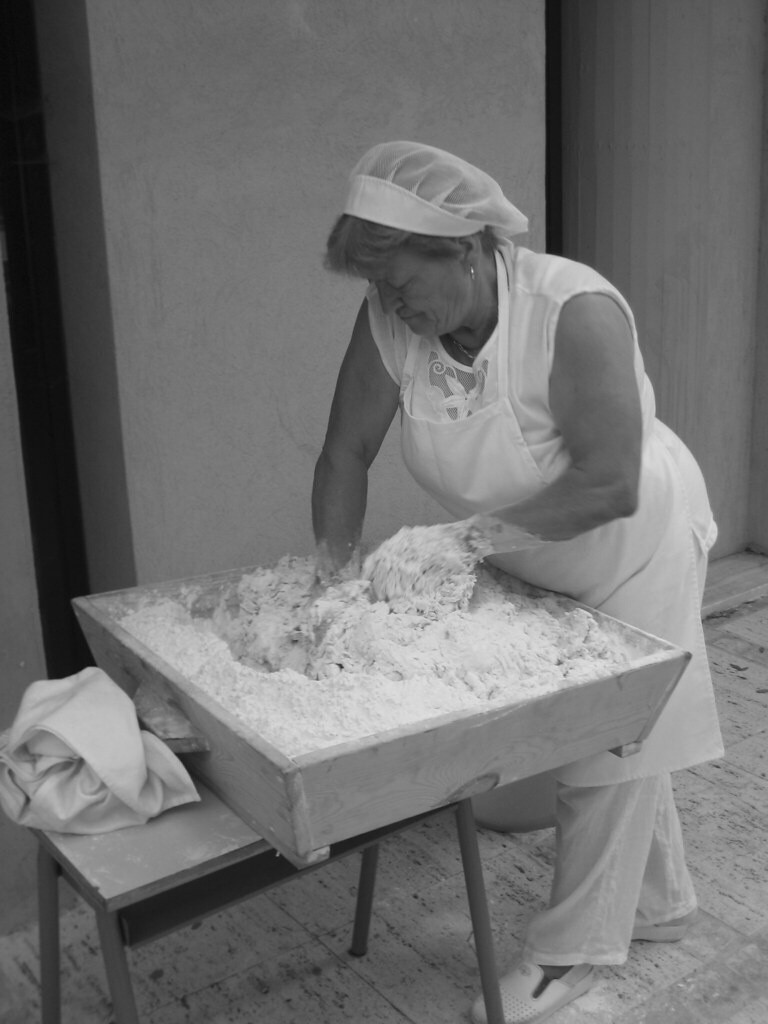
[632,900,697,943]
[470,961,594,1024]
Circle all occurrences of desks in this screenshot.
[25,769,506,1024]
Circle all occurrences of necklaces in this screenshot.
[453,339,475,360]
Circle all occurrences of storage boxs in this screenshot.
[65,542,694,868]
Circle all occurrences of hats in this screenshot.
[342,141,529,238]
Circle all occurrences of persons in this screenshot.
[311,140,726,1024]
